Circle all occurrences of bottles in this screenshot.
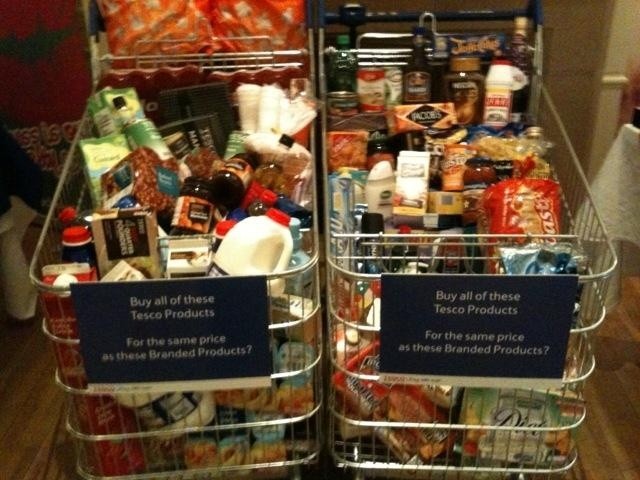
[58,208,91,229]
[403,27,431,103]
[328,34,357,92]
[113,95,173,162]
[240,134,296,208]
[508,17,535,135]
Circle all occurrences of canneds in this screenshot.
[213,153,258,209]
[171,175,215,234]
[326,66,405,114]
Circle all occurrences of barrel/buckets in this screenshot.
[204,207,294,311]
[112,392,219,445]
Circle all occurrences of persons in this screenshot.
[1,124,61,328]
